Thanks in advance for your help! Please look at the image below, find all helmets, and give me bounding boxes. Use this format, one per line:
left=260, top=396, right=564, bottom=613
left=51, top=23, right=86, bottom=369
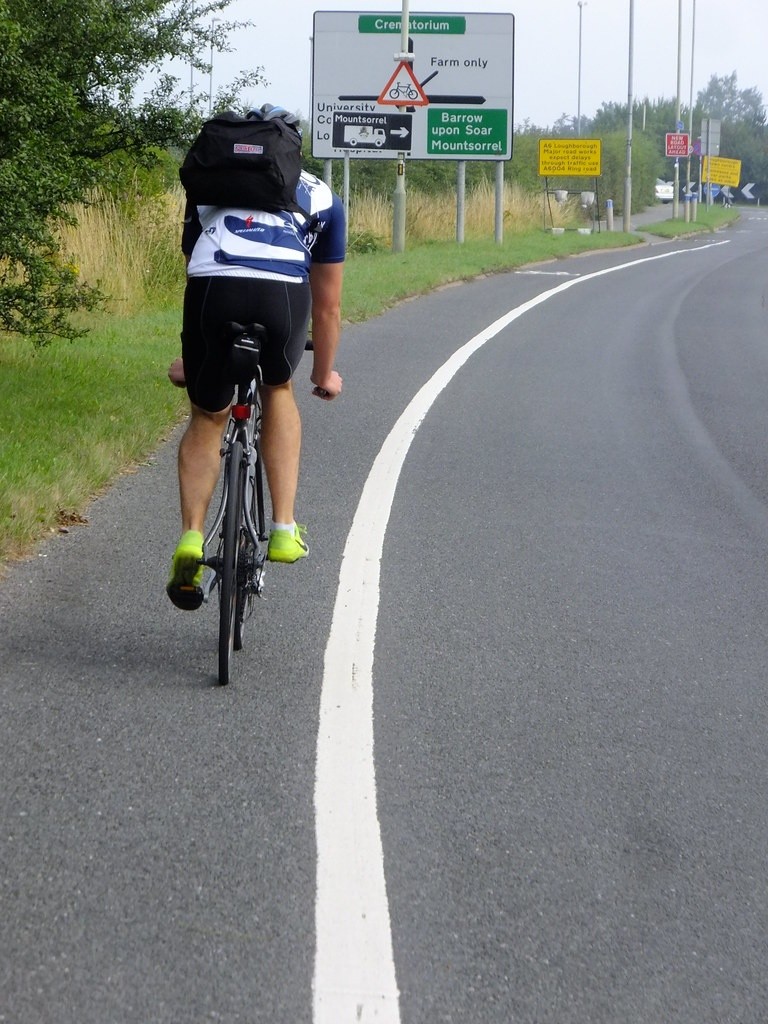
left=246, top=104, right=301, bottom=134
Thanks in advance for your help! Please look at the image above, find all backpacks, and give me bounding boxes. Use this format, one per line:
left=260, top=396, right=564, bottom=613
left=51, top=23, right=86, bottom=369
left=178, top=111, right=322, bottom=257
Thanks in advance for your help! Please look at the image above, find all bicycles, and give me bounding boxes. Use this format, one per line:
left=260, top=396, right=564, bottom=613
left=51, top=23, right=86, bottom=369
left=169, top=339, right=330, bottom=687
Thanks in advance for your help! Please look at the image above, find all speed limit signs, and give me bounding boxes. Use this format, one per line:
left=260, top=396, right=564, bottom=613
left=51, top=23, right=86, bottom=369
left=704, top=182, right=720, bottom=197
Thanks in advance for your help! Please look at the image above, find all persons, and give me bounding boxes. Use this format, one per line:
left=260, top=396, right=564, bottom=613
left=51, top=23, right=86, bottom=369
left=166, top=105, right=345, bottom=613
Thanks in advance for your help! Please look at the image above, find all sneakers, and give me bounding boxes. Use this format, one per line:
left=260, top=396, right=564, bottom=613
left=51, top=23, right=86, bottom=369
left=267, top=525, right=310, bottom=563
left=165, top=531, right=204, bottom=610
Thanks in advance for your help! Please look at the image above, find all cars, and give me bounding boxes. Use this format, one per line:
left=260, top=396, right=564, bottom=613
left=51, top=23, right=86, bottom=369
left=655, top=181, right=674, bottom=204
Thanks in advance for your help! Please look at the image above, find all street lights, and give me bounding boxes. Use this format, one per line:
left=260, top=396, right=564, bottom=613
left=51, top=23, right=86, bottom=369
left=578, top=1, right=587, bottom=138
